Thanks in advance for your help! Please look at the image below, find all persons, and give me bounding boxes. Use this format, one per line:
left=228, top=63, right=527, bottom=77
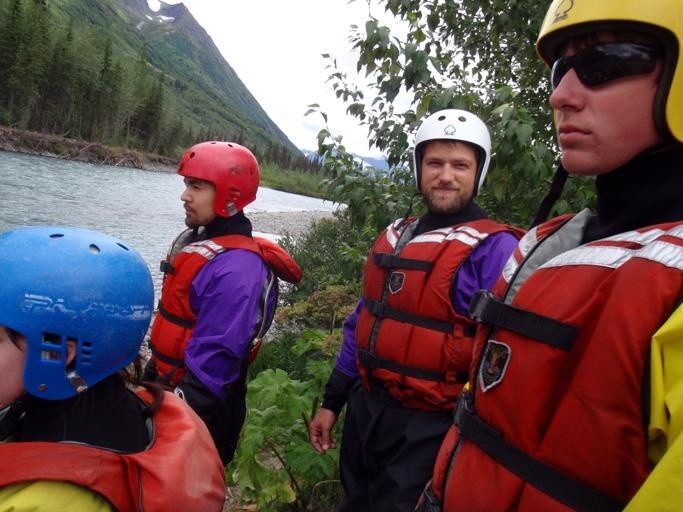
left=0, top=221, right=231, bottom=511
left=146, top=138, right=305, bottom=479
left=305, top=106, right=528, bottom=511
left=415, top=1, right=683, bottom=512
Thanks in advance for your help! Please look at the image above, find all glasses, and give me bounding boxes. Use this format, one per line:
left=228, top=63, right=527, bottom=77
left=544, top=39, right=665, bottom=90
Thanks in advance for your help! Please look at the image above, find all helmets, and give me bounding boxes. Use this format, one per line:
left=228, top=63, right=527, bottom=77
left=535, top=0, right=683, bottom=158
left=412, top=108, right=491, bottom=203
left=176, top=140, right=260, bottom=220
left=0, top=225, right=155, bottom=401
